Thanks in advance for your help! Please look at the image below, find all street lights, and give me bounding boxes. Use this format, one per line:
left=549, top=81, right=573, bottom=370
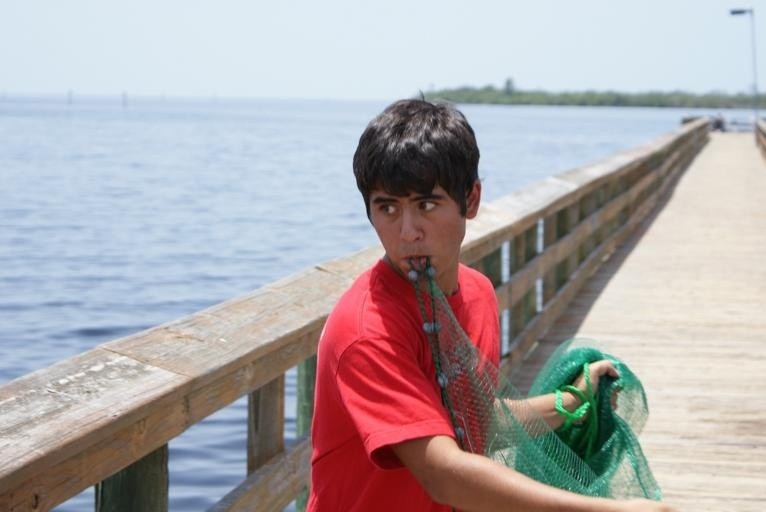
left=728, top=5, right=762, bottom=116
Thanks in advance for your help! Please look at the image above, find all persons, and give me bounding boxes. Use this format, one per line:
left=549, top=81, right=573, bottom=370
left=305, top=98, right=679, bottom=511
